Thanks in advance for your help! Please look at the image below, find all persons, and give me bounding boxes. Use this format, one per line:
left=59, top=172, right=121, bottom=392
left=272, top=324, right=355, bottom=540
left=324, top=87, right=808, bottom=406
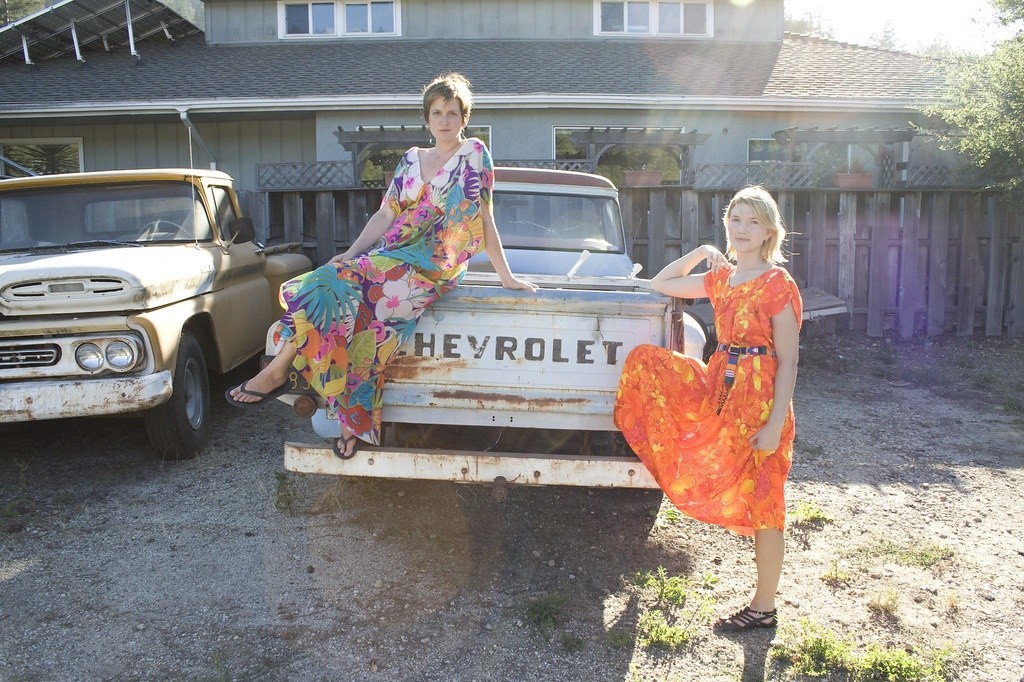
left=621, top=184, right=803, bottom=634
left=224, top=73, right=541, bottom=458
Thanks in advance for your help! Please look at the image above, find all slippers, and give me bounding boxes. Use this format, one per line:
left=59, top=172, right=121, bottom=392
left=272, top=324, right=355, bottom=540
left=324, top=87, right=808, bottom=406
left=333, top=433, right=357, bottom=459
left=224, top=373, right=292, bottom=409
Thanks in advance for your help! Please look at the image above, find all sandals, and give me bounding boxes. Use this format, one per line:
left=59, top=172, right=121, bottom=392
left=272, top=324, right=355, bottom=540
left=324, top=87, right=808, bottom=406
left=713, top=605, right=778, bottom=631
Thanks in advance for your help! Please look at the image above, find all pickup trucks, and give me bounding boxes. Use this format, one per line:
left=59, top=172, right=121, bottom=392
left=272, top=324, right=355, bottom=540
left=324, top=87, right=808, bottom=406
left=262, top=168, right=707, bottom=492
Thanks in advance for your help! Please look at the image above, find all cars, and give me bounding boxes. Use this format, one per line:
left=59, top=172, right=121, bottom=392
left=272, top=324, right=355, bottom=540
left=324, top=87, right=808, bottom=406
left=0, top=164, right=313, bottom=459
